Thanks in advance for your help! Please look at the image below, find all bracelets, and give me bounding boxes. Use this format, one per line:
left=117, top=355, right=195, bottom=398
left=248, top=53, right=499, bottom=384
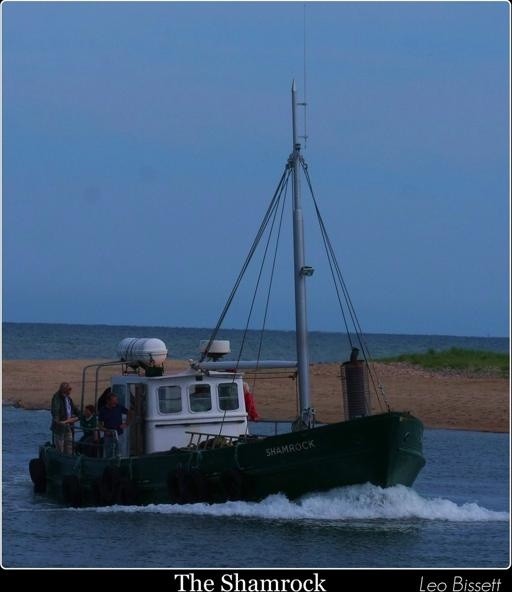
left=126, top=421, right=129, bottom=425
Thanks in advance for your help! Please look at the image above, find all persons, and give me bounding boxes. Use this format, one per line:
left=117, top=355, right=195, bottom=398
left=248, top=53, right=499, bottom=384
left=243, top=382, right=261, bottom=421
left=78, top=404, right=100, bottom=456
left=49, top=382, right=81, bottom=456
left=99, top=391, right=131, bottom=458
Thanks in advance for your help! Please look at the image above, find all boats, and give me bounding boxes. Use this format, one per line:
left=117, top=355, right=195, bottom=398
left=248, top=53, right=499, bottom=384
left=31, top=77, right=430, bottom=509
